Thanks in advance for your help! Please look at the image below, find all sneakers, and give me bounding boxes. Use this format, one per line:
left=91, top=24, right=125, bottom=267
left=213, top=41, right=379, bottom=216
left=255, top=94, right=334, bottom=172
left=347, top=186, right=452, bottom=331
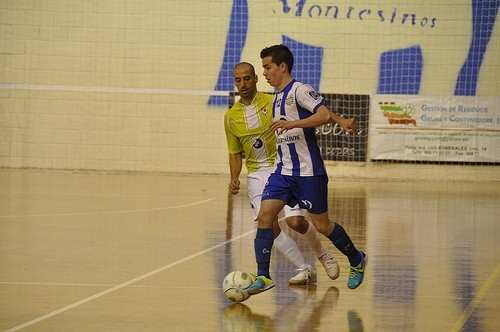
left=247, top=272, right=276, bottom=295
left=288, top=266, right=318, bottom=285
left=318, top=247, right=340, bottom=280
left=347, top=250, right=369, bottom=289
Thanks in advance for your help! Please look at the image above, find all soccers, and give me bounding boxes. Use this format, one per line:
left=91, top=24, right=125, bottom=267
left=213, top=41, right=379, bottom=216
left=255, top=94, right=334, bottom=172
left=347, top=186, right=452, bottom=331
left=223, top=303, right=251, bottom=330
left=222, top=270, right=253, bottom=303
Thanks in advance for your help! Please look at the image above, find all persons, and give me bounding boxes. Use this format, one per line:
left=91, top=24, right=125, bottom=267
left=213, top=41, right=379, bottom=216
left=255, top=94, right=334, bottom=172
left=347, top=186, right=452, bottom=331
left=224, top=62, right=357, bottom=284
left=248, top=44, right=368, bottom=295
